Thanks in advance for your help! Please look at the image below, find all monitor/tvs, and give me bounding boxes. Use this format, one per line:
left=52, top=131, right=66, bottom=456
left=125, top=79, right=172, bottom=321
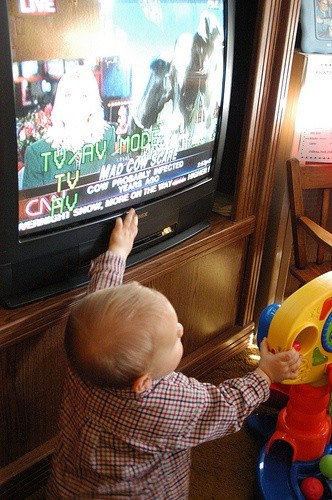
left=0, top=0, right=236, bottom=310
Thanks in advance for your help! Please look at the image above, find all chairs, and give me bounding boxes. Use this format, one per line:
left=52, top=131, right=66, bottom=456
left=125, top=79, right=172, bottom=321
left=286, top=157, right=332, bottom=285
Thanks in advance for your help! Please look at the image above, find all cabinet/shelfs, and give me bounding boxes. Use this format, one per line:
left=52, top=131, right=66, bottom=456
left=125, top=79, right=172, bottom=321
left=0, top=215, right=302, bottom=500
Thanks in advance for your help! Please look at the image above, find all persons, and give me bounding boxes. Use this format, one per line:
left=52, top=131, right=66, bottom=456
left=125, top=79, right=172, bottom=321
left=317, top=0, right=332, bottom=38
left=22, top=65, right=115, bottom=190
left=48, top=208, right=303, bottom=500
